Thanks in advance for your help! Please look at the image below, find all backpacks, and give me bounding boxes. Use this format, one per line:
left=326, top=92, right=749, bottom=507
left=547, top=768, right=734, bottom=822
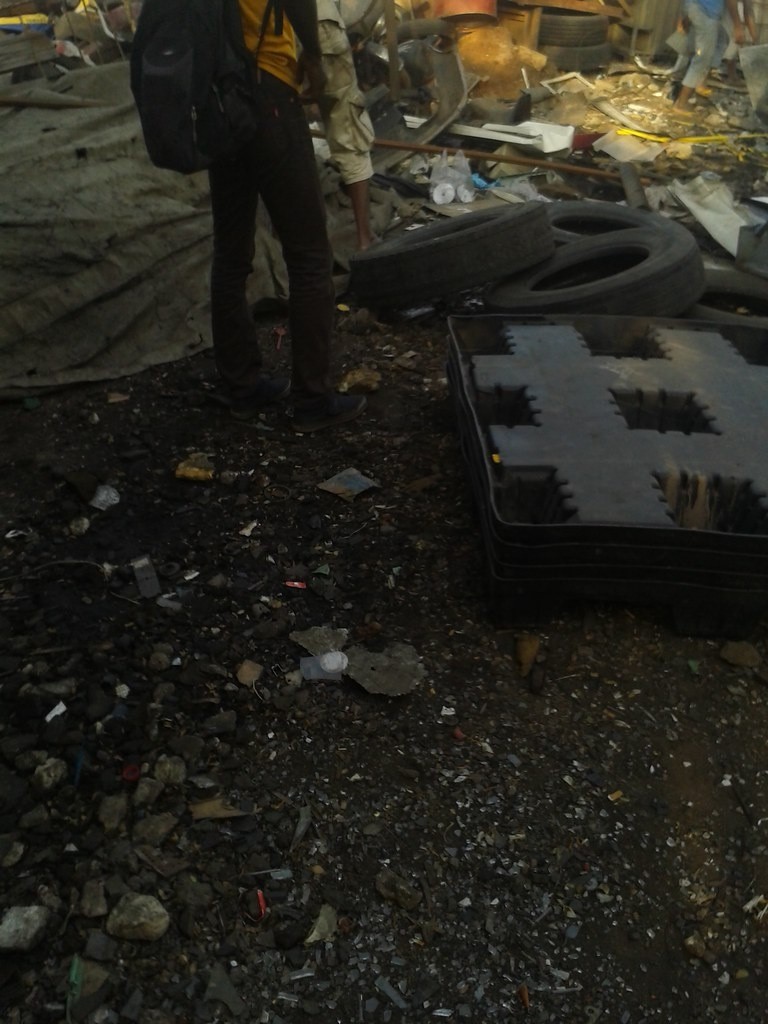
left=130, top=0, right=264, bottom=175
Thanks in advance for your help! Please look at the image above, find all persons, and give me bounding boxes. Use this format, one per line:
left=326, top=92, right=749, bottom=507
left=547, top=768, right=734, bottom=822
left=169, top=0, right=375, bottom=435
left=663, top=0, right=763, bottom=116
left=297, top=1, right=386, bottom=260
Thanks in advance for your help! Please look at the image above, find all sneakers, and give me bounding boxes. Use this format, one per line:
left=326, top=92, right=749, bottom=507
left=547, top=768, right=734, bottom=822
left=292, top=394, right=367, bottom=434
left=231, top=376, right=292, bottom=417
